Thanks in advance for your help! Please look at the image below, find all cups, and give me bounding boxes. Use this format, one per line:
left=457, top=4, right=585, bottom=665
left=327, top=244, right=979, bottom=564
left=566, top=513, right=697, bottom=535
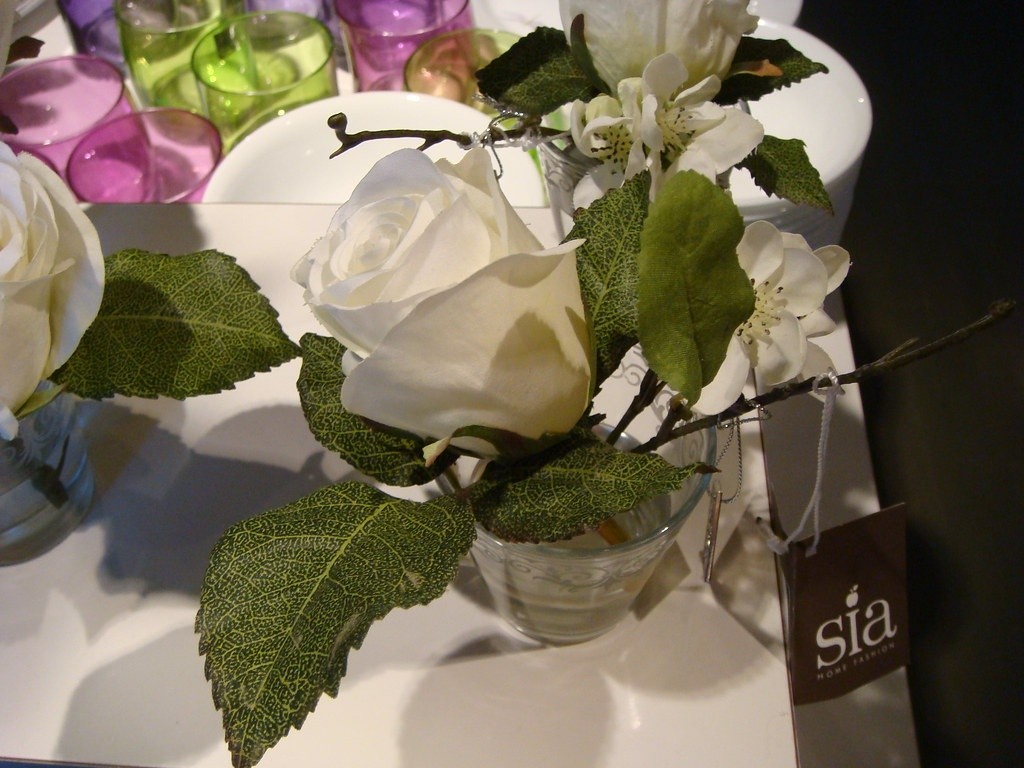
left=113, top=0, right=259, bottom=148
left=67, top=107, right=222, bottom=205
left=718, top=21, right=874, bottom=254
left=401, top=28, right=544, bottom=132
left=190, top=10, right=340, bottom=159
left=333, top=0, right=474, bottom=95
left=245, top=0, right=335, bottom=58
left=54, top=0, right=135, bottom=80
left=0, top=55, right=149, bottom=206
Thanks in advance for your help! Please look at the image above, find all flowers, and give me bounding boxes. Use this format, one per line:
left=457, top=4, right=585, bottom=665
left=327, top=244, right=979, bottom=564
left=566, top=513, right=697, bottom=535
left=0, top=137, right=305, bottom=509
left=188, top=3, right=1013, bottom=766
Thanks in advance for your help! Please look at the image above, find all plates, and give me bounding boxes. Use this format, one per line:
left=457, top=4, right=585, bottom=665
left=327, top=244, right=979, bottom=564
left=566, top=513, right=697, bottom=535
left=201, top=91, right=548, bottom=207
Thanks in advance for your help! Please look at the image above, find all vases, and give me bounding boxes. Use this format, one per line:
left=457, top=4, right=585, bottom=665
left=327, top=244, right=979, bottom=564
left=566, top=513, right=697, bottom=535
left=437, top=343, right=719, bottom=644
left=0, top=383, right=95, bottom=569
